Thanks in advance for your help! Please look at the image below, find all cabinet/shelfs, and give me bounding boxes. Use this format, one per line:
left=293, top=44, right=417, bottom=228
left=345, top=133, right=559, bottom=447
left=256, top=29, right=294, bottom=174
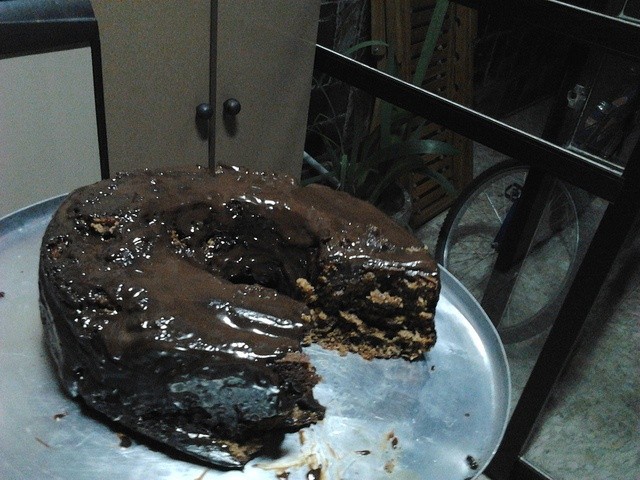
left=88, top=0, right=320, bottom=183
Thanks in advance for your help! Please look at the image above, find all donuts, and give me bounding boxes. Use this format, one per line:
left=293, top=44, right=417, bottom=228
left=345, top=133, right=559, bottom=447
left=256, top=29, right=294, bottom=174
left=43, top=166, right=441, bottom=469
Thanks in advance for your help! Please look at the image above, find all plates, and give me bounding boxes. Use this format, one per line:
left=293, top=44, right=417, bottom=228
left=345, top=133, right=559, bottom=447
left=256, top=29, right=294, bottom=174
left=0, top=191, right=512, bottom=479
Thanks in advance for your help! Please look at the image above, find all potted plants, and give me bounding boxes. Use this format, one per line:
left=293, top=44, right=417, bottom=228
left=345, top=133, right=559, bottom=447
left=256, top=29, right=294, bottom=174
left=307, top=0, right=464, bottom=237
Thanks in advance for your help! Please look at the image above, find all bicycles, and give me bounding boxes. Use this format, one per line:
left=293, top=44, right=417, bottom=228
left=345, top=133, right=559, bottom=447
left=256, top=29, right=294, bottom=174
left=435, top=78, right=628, bottom=344
